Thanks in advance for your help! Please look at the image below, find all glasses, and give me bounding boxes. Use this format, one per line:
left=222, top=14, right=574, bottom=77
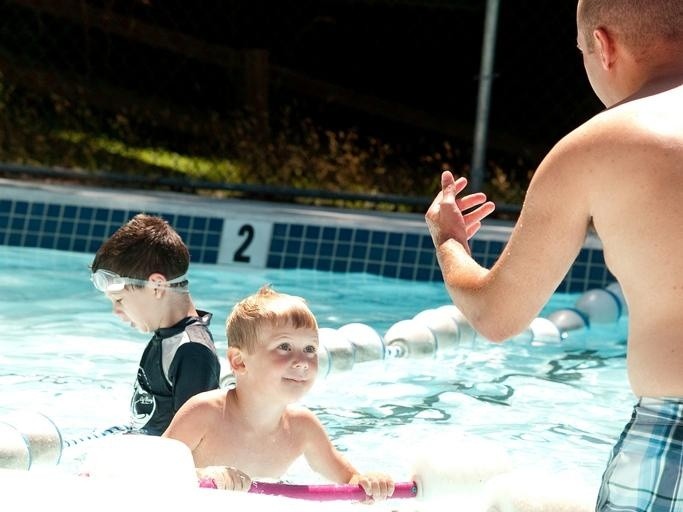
left=89, top=268, right=190, bottom=294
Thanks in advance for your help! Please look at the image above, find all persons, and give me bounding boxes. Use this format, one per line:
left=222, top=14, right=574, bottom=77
left=89, top=212, right=221, bottom=437
left=161, top=284, right=396, bottom=506
left=423, top=0, right=683, bottom=512
